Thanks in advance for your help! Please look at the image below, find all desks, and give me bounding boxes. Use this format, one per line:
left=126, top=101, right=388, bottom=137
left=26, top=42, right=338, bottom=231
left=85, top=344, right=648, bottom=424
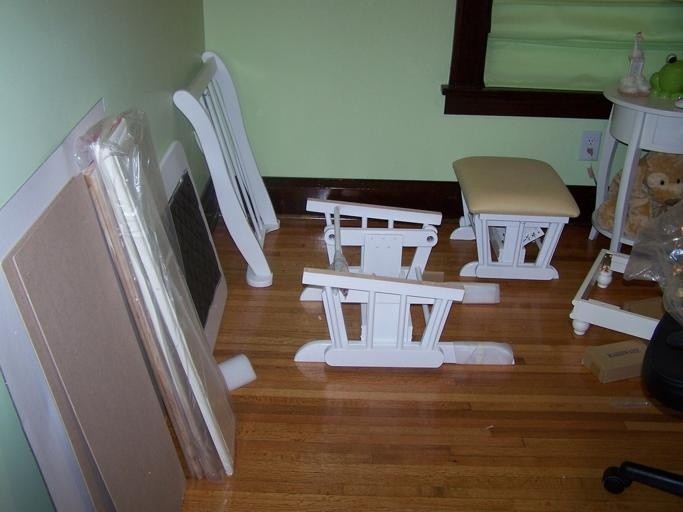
left=588, top=86, right=683, bottom=253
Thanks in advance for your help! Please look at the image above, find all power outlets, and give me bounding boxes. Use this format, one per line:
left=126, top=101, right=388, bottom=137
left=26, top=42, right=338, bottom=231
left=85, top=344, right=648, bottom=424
left=578, top=130, right=602, bottom=160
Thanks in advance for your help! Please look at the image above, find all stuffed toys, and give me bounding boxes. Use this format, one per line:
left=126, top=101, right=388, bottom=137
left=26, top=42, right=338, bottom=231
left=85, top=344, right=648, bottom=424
left=595, top=152, right=683, bottom=238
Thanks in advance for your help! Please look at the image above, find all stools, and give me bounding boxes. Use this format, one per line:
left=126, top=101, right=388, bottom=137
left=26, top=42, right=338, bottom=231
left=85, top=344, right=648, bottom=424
left=451, top=156, right=580, bottom=280
left=604, top=311, right=683, bottom=501
